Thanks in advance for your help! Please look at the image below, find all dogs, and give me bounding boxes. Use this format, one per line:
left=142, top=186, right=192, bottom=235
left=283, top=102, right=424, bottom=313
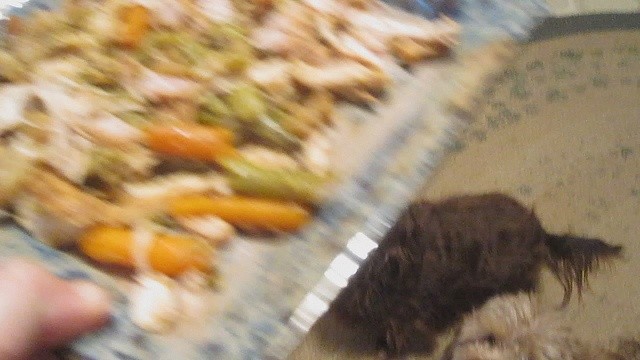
left=446, top=292, right=640, bottom=360
left=329, top=192, right=624, bottom=360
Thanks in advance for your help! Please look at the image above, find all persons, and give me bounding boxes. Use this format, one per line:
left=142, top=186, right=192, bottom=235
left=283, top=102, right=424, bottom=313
left=0, top=259, right=115, bottom=360
